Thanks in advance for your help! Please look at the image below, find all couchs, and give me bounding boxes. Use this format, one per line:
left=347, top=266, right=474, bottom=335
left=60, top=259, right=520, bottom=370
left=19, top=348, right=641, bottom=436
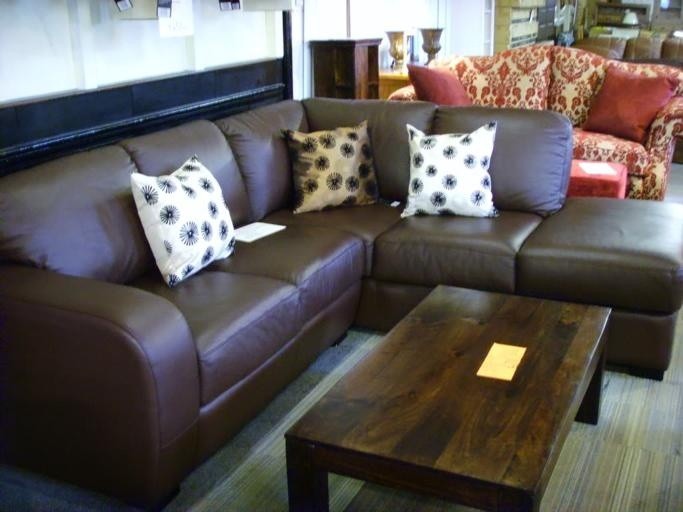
left=0, top=94, right=682, bottom=512
left=384, top=45, right=682, bottom=199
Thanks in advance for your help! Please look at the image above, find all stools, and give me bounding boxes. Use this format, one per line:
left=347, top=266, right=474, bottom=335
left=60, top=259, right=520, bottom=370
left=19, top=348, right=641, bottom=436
left=562, top=158, right=628, bottom=199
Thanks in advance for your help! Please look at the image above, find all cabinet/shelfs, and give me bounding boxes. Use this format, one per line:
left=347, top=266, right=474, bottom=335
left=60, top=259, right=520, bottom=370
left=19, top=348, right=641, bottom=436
left=306, top=36, right=384, bottom=102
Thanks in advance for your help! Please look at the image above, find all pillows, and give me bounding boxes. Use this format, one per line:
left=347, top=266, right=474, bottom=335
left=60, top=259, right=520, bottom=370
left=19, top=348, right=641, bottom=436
left=582, top=65, right=682, bottom=142
left=278, top=119, right=385, bottom=216
left=121, top=154, right=238, bottom=289
left=406, top=63, right=474, bottom=106
left=399, top=114, right=503, bottom=220
